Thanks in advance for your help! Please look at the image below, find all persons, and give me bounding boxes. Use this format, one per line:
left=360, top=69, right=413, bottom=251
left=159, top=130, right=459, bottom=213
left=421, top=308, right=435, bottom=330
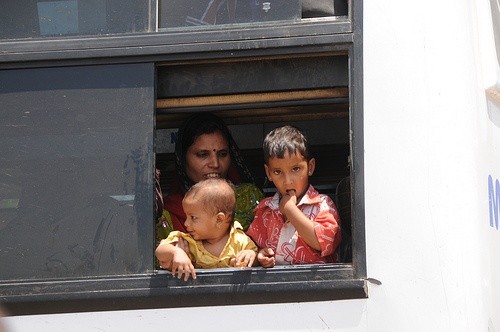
left=245, top=125, right=342, bottom=269
left=155, top=177, right=258, bottom=282
left=154, top=116, right=262, bottom=266
